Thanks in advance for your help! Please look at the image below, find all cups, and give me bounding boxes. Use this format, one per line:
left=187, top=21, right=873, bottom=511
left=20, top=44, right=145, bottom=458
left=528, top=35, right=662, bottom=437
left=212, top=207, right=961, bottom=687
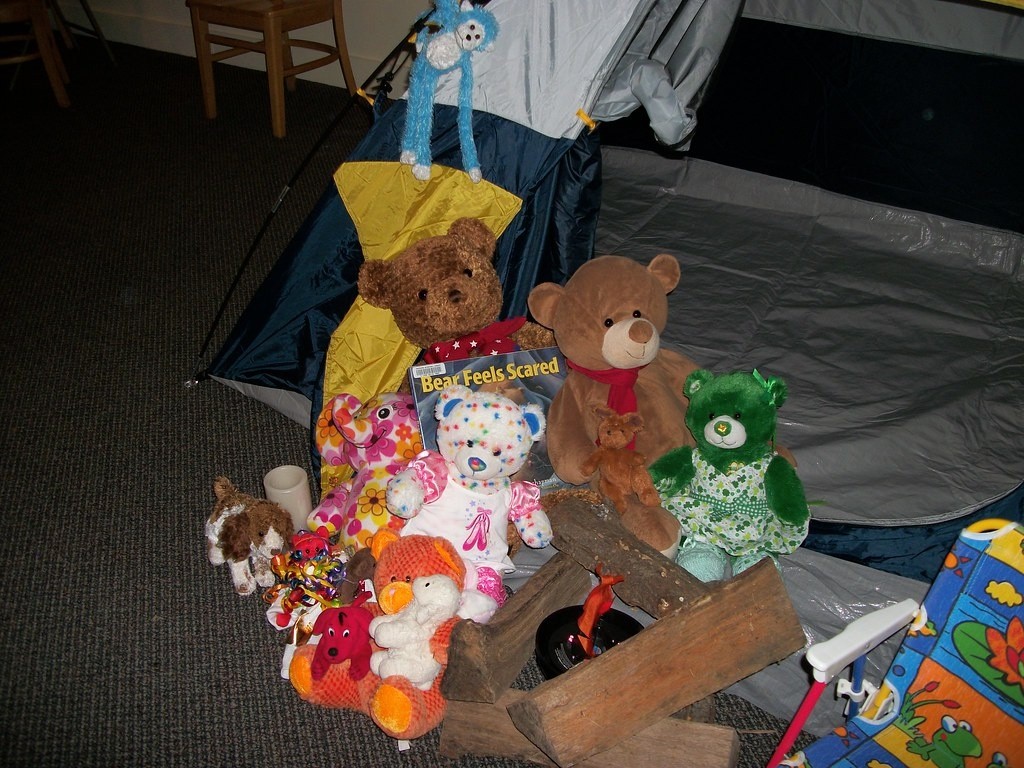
left=264, top=465, right=313, bottom=534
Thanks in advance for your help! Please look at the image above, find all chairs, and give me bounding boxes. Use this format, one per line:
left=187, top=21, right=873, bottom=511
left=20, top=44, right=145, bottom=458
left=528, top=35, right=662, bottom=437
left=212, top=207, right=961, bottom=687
left=764, top=516, right=1024, bottom=768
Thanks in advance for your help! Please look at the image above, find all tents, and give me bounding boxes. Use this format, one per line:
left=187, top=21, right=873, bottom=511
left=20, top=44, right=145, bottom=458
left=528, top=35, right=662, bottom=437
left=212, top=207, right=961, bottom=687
left=184, top=0, right=1024, bottom=737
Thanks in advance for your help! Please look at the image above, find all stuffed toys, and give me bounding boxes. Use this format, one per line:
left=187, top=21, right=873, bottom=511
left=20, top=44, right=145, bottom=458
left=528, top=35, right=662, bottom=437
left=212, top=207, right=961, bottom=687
left=399, top=0, right=499, bottom=183
left=386, top=385, right=553, bottom=625
left=528, top=254, right=795, bottom=551
left=645, top=369, right=811, bottom=583
left=357, top=217, right=558, bottom=395
left=580, top=403, right=661, bottom=516
left=204, top=476, right=294, bottom=596
left=262, top=394, right=466, bottom=740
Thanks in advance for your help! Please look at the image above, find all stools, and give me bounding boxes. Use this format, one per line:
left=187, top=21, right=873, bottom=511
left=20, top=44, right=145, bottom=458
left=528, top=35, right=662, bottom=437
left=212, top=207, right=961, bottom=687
left=0, top=0, right=118, bottom=108
left=186, top=0, right=357, bottom=138
